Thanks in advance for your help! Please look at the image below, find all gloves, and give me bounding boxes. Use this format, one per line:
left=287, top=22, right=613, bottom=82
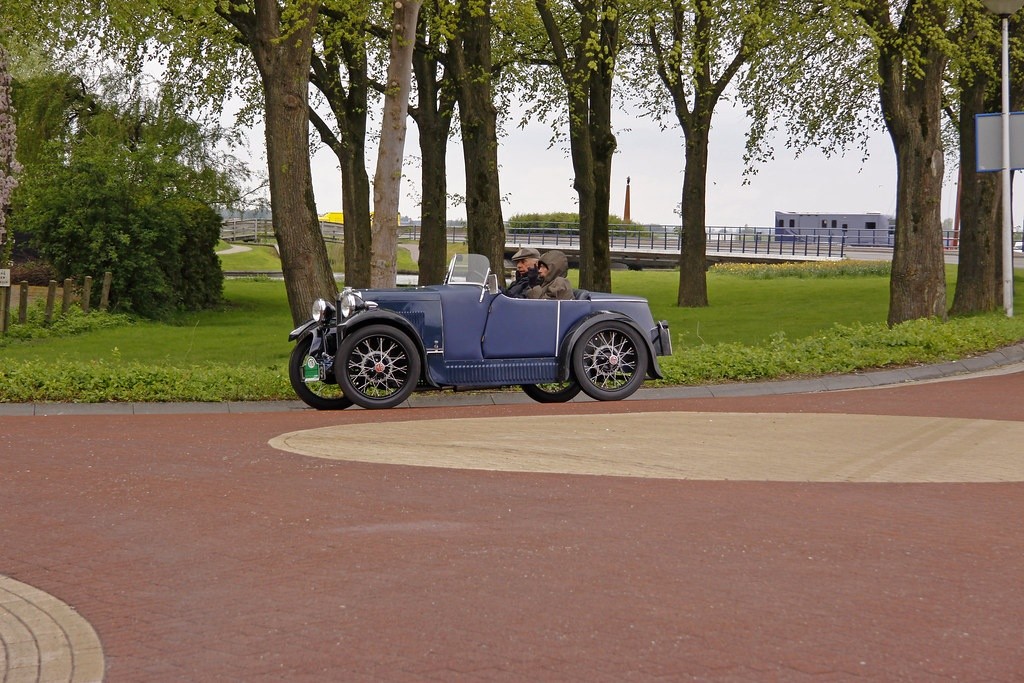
left=527, top=263, right=538, bottom=287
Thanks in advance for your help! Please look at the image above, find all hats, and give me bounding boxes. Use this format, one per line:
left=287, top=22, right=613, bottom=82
left=512, top=248, right=541, bottom=261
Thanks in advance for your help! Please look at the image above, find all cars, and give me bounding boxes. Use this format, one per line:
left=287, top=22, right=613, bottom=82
left=284, top=251, right=674, bottom=409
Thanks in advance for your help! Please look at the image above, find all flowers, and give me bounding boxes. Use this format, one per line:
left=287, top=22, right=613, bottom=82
left=0, top=42, right=24, bottom=270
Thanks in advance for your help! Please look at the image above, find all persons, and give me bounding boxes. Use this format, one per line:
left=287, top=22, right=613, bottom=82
left=488, top=248, right=540, bottom=298
left=521, top=250, right=575, bottom=300
